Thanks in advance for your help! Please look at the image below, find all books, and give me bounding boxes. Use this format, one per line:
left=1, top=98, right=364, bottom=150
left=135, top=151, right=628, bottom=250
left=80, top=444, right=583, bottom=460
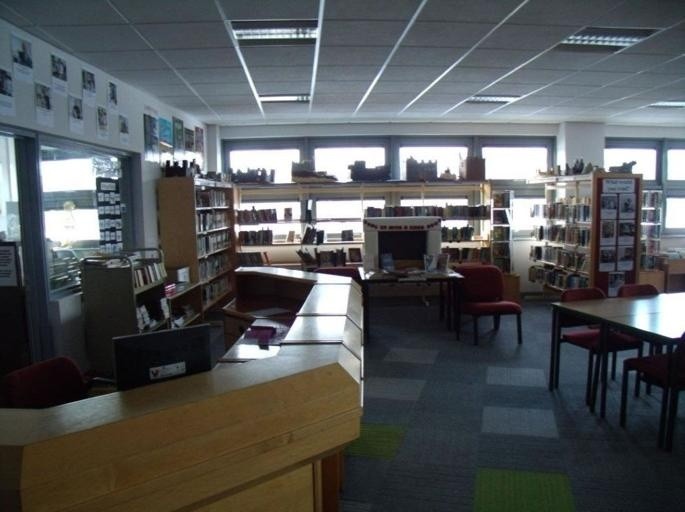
left=442, top=246, right=490, bottom=261
left=342, top=230, right=352, bottom=239
left=121, top=261, right=177, bottom=330
left=493, top=189, right=512, bottom=273
left=237, top=208, right=276, bottom=266
left=288, top=230, right=295, bottom=242
left=442, top=227, right=472, bottom=240
left=285, top=208, right=292, bottom=218
left=198, top=188, right=232, bottom=303
left=302, top=227, right=325, bottom=245
left=296, top=248, right=362, bottom=267
left=363, top=207, right=492, bottom=220
left=530, top=203, right=590, bottom=292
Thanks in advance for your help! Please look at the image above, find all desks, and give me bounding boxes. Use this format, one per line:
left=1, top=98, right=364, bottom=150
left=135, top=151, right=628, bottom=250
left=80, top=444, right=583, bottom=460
left=658, top=256, right=685, bottom=293
left=355, top=267, right=466, bottom=342
left=549, top=292, right=685, bottom=451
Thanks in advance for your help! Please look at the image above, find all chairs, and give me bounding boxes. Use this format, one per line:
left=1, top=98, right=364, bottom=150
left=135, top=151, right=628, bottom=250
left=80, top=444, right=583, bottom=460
left=619, top=331, right=685, bottom=454
left=610, top=283, right=674, bottom=396
left=453, top=264, right=523, bottom=345
left=315, top=267, right=370, bottom=341
left=0, top=355, right=117, bottom=409
left=554, top=286, right=643, bottom=406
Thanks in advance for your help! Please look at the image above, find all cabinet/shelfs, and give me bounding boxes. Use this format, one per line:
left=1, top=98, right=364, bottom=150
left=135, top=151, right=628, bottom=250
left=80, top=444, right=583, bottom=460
left=79, top=247, right=171, bottom=378
left=165, top=281, right=203, bottom=329
left=528, top=167, right=665, bottom=298
left=235, top=181, right=514, bottom=273
left=157, top=175, right=236, bottom=322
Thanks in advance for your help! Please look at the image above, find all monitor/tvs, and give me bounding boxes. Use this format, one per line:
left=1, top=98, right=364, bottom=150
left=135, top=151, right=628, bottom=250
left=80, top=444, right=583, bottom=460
left=111, top=318, right=227, bottom=392
